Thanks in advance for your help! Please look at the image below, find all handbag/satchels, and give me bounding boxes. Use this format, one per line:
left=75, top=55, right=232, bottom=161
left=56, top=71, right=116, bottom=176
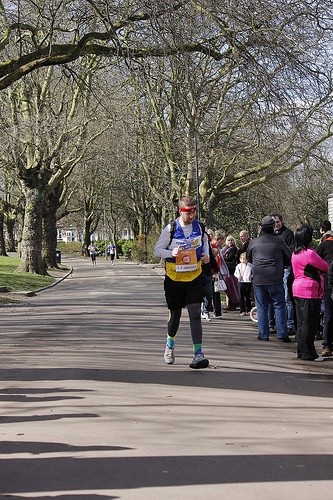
left=214, top=276, right=228, bottom=293
left=223, top=276, right=239, bottom=304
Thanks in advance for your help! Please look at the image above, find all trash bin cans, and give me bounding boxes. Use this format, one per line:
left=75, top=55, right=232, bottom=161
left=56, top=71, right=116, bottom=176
left=55, top=251, right=61, bottom=263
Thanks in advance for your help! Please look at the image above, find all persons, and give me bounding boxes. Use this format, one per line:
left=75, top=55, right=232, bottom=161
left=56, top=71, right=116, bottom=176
left=246, top=216, right=293, bottom=342
left=107, top=241, right=115, bottom=266
left=233, top=252, right=253, bottom=315
left=221, top=235, right=238, bottom=312
left=269, top=213, right=294, bottom=335
left=239, top=230, right=251, bottom=251
left=201, top=232, right=213, bottom=321
left=88, top=241, right=98, bottom=266
left=319, top=220, right=333, bottom=246
left=315, top=234, right=333, bottom=356
left=153, top=196, right=210, bottom=369
left=291, top=225, right=329, bottom=362
left=201, top=229, right=229, bottom=322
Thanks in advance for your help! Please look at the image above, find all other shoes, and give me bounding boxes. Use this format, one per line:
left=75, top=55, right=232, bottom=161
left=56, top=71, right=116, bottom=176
left=241, top=312, right=246, bottom=316
left=201, top=312, right=209, bottom=318
left=247, top=311, right=249, bottom=315
left=322, top=346, right=332, bottom=355
left=314, top=357, right=324, bottom=361
left=277, top=335, right=291, bottom=342
left=257, top=335, right=269, bottom=340
left=214, top=313, right=222, bottom=319
left=224, top=306, right=235, bottom=311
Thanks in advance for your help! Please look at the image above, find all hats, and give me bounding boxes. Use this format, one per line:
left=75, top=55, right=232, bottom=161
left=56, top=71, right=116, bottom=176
left=259, top=216, right=275, bottom=227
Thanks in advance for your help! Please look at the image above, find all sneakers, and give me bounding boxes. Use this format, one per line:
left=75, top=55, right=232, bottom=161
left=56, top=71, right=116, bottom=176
left=190, top=354, right=208, bottom=369
left=164, top=345, right=174, bottom=364
